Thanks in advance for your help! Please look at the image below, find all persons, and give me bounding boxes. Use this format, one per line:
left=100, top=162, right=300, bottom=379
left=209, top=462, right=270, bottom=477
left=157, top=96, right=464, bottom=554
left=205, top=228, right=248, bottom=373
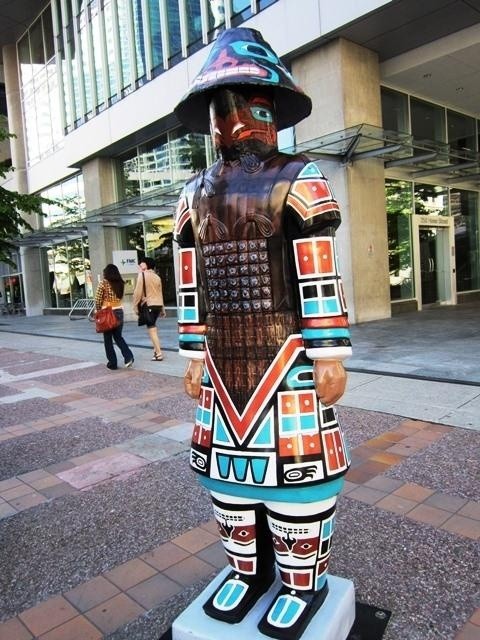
left=93, top=263, right=135, bottom=370
left=162, top=27, right=353, bottom=640
left=132, top=257, right=166, bottom=362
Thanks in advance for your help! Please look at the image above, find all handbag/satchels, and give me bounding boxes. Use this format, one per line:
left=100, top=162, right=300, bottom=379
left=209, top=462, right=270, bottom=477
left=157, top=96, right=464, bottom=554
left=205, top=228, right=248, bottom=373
left=138, top=300, right=151, bottom=326
left=93, top=307, right=119, bottom=333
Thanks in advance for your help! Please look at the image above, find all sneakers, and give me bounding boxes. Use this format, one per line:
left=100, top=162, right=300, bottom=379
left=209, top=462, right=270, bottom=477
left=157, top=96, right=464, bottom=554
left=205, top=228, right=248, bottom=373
left=125, top=359, right=134, bottom=368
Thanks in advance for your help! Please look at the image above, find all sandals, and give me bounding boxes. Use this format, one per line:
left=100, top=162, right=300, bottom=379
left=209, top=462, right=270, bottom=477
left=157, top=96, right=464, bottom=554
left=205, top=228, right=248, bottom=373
left=152, top=352, right=163, bottom=361
